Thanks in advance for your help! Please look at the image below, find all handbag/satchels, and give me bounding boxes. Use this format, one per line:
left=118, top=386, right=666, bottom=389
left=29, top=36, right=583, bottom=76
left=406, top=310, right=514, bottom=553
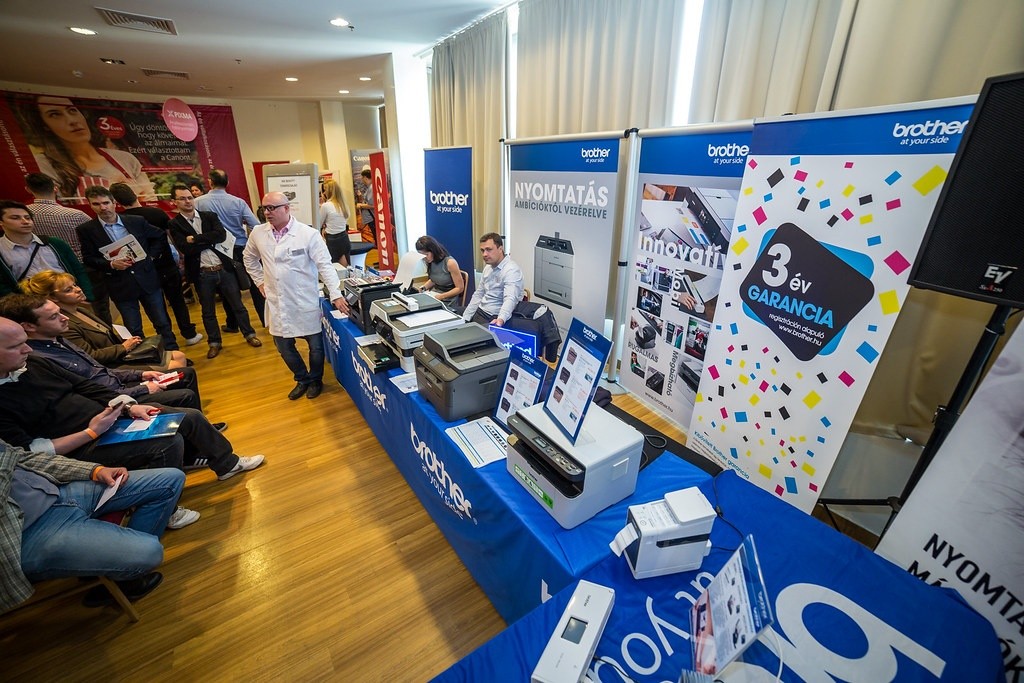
left=124, top=335, right=167, bottom=367
left=230, top=256, right=252, bottom=291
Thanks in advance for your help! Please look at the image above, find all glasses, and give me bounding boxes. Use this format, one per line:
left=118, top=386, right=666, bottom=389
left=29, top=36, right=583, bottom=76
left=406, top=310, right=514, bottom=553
left=175, top=196, right=194, bottom=201
left=262, top=204, right=288, bottom=212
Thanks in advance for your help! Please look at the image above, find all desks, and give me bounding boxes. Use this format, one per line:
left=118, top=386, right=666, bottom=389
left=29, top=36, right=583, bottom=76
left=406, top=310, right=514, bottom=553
left=321, top=293, right=724, bottom=581
left=427, top=470, right=1005, bottom=683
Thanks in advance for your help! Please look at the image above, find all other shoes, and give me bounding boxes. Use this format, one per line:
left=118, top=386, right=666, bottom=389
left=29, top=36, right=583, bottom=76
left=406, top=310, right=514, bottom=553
left=212, top=422, right=228, bottom=433
left=221, top=324, right=239, bottom=333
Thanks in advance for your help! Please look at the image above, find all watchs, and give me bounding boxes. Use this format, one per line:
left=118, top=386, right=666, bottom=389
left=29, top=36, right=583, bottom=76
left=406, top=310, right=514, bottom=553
left=192, top=236, right=195, bottom=243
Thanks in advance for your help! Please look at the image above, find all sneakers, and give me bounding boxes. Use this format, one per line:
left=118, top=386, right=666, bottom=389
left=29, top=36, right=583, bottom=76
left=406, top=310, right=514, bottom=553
left=184, top=457, right=210, bottom=468
left=219, top=454, right=265, bottom=480
left=168, top=508, right=200, bottom=528
left=185, top=333, right=204, bottom=345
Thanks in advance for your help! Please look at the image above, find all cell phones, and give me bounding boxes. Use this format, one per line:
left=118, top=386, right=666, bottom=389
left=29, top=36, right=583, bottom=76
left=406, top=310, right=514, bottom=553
left=112, top=401, right=123, bottom=410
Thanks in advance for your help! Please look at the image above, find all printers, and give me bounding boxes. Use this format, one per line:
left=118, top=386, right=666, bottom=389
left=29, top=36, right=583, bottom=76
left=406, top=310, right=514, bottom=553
left=675, top=361, right=704, bottom=405
left=553, top=346, right=577, bottom=403
left=533, top=232, right=574, bottom=310
left=342, top=280, right=512, bottom=422
left=639, top=184, right=737, bottom=271
left=501, top=368, right=518, bottom=412
left=635, top=325, right=656, bottom=349
left=506, top=399, right=645, bottom=529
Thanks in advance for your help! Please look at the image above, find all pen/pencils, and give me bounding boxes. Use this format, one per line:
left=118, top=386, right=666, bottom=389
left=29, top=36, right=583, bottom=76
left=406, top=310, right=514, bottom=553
left=159, top=372, right=184, bottom=384
left=132, top=409, right=161, bottom=419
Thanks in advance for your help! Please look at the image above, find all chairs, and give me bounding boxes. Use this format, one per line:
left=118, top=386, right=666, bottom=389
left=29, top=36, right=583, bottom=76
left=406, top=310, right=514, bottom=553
left=0, top=508, right=139, bottom=636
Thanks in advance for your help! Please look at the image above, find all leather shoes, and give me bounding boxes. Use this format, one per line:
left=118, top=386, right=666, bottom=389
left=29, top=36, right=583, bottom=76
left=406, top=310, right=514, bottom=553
left=205, top=344, right=222, bottom=359
left=84, top=572, right=164, bottom=607
left=288, top=381, right=308, bottom=399
left=245, top=335, right=261, bottom=347
left=309, top=381, right=325, bottom=398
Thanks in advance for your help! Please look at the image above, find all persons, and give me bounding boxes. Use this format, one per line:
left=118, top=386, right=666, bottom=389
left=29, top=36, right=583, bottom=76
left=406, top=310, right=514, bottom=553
left=416, top=235, right=464, bottom=309
left=0, top=201, right=94, bottom=305
left=0, top=270, right=265, bottom=606
left=22, top=172, right=114, bottom=335
left=641, top=290, right=657, bottom=310
left=631, top=352, right=641, bottom=371
left=74, top=181, right=203, bottom=366
left=674, top=292, right=697, bottom=309
left=243, top=192, right=350, bottom=400
left=21, top=93, right=160, bottom=201
left=461, top=232, right=524, bottom=330
left=356, top=169, right=376, bottom=249
left=317, top=181, right=351, bottom=269
left=166, top=168, right=261, bottom=359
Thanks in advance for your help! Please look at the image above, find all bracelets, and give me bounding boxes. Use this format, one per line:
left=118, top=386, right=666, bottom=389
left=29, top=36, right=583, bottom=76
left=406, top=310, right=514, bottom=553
left=122, top=402, right=135, bottom=417
left=93, top=465, right=106, bottom=481
left=83, top=428, right=98, bottom=439
left=420, top=286, right=426, bottom=291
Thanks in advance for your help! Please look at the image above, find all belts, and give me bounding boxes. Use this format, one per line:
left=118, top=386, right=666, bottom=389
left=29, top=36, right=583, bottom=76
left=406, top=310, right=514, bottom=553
left=199, top=262, right=224, bottom=272
